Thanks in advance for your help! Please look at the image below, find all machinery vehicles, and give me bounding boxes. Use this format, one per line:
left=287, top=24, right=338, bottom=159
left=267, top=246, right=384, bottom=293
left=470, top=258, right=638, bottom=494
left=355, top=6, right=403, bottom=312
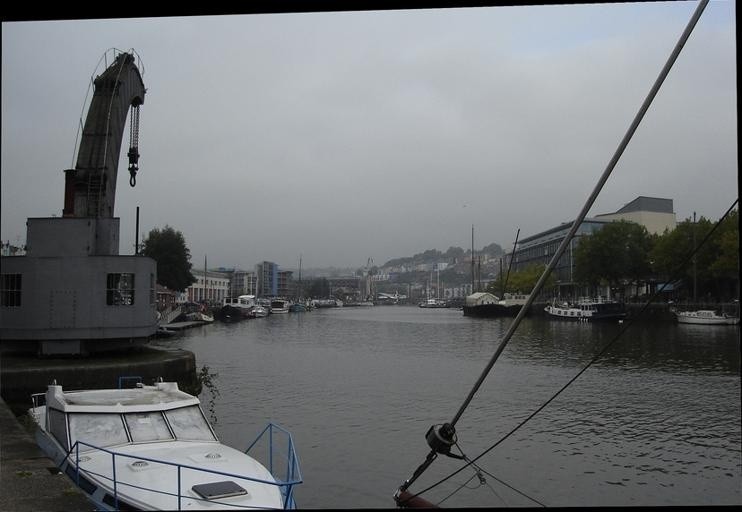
left=0, top=47, right=159, bottom=358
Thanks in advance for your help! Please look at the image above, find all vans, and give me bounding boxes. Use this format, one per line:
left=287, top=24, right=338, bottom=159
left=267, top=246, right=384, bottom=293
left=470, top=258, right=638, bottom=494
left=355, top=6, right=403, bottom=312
left=223, top=296, right=254, bottom=307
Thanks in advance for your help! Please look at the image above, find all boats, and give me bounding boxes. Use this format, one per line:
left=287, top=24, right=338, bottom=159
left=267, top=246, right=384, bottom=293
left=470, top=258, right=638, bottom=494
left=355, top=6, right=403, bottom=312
left=460, top=291, right=741, bottom=326
left=251, top=300, right=303, bottom=317
left=27, top=375, right=304, bottom=512
left=418, top=298, right=449, bottom=308
